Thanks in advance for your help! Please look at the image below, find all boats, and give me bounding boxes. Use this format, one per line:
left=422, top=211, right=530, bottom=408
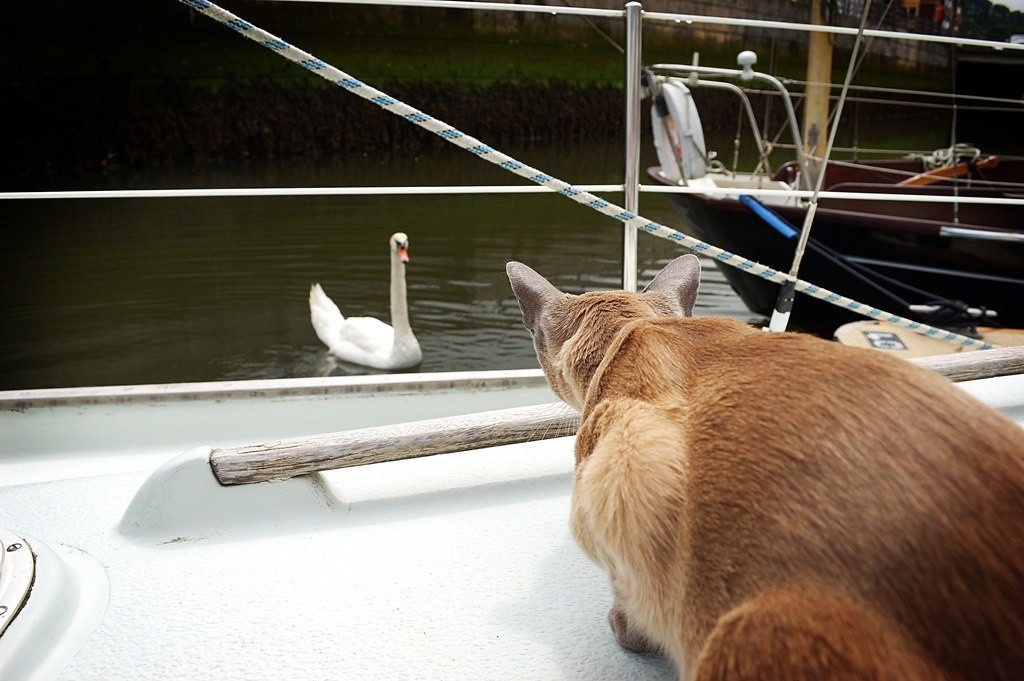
left=646, top=48, right=1023, bottom=340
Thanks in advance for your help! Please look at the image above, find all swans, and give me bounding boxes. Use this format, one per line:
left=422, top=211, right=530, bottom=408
left=308, top=232, right=424, bottom=369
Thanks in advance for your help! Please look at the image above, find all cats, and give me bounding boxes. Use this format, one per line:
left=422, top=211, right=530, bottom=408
left=506, top=255, right=1024, bottom=681
